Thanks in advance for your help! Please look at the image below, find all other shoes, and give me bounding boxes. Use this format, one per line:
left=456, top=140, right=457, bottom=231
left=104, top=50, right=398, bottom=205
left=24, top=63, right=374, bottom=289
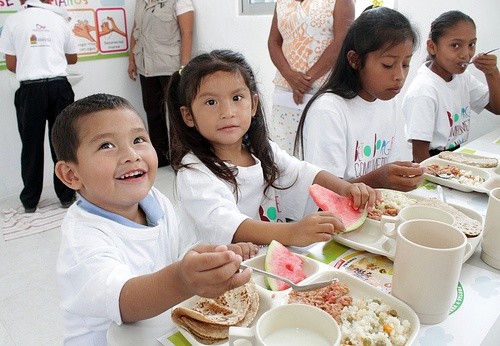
left=25, top=207, right=36, bottom=213
left=61, top=199, right=77, bottom=209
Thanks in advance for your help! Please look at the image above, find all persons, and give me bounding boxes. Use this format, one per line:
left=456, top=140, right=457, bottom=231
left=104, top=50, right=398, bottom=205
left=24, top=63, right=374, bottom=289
left=0, top=0, right=80, bottom=213
left=128, top=0, right=196, bottom=168
left=52, top=94, right=260, bottom=346
left=404, top=11, right=500, bottom=164
left=268, top=0, right=355, bottom=160
left=165, top=47, right=381, bottom=254
left=294, top=0, right=426, bottom=193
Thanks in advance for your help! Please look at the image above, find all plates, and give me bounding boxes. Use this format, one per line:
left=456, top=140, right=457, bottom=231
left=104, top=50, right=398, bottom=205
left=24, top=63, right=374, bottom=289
left=171, top=252, right=420, bottom=346
left=416, top=151, right=500, bottom=195
left=331, top=188, right=485, bottom=264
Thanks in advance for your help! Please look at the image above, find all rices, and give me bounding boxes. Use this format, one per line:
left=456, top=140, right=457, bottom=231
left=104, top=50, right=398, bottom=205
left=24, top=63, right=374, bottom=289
left=338, top=299, right=411, bottom=346
left=376, top=188, right=411, bottom=211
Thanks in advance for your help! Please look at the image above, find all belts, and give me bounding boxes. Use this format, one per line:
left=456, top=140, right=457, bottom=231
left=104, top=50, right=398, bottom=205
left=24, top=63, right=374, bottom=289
left=22, top=77, right=66, bottom=85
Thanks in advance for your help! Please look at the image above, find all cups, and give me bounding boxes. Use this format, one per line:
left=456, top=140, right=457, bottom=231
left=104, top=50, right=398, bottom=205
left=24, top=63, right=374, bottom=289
left=480, top=187, right=500, bottom=270
left=381, top=205, right=456, bottom=255
left=228, top=303, right=342, bottom=346
left=390, top=219, right=465, bottom=325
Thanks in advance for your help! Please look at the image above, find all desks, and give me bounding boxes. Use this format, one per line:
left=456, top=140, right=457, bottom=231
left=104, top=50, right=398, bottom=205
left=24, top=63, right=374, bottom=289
left=105, top=127, right=500, bottom=345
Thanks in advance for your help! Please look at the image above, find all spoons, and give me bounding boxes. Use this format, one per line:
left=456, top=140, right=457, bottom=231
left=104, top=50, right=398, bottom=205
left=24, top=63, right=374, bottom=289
left=458, top=48, right=500, bottom=67
left=238, top=264, right=337, bottom=292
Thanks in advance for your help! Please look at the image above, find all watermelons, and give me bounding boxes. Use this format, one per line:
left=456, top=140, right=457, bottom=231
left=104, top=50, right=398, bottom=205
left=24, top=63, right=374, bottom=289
left=264, top=240, right=307, bottom=291
left=308, top=183, right=369, bottom=232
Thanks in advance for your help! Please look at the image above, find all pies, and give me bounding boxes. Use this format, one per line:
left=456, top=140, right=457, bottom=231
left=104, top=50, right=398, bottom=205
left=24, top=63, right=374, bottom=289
left=171, top=276, right=259, bottom=342
left=418, top=199, right=482, bottom=237
left=439, top=151, right=498, bottom=167
